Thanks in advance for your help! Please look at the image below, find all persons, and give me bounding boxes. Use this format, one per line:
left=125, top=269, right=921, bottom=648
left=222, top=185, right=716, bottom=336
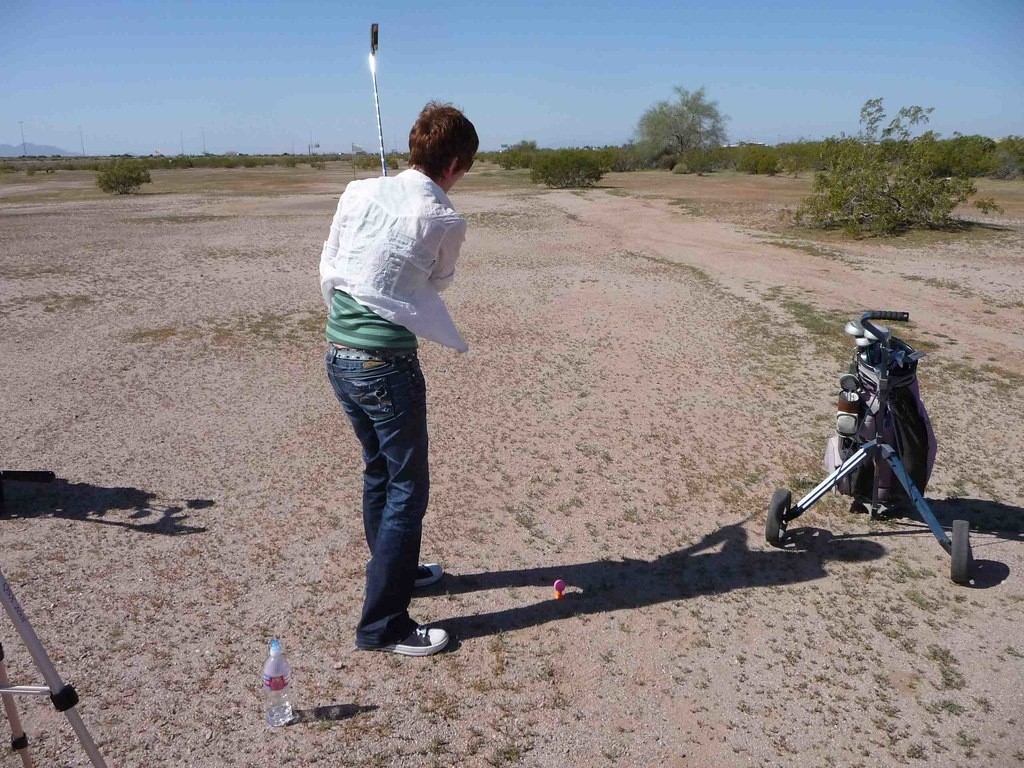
left=320, top=102, right=479, bottom=656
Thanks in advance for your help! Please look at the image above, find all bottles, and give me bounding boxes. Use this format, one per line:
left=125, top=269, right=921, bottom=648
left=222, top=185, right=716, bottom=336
left=262, top=640, right=293, bottom=727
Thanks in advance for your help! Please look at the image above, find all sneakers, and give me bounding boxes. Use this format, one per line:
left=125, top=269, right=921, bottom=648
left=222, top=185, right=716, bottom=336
left=373, top=624, right=449, bottom=657
left=414, top=564, right=443, bottom=586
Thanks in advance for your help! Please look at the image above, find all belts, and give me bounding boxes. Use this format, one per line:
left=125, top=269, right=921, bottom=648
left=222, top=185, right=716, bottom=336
left=327, top=342, right=411, bottom=361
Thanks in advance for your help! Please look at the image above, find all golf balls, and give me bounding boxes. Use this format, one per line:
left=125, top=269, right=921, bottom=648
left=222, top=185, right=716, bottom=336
left=554, top=579, right=566, bottom=591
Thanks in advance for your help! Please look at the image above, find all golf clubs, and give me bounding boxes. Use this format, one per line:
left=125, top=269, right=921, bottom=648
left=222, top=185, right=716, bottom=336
left=370, top=22, right=388, bottom=177
left=837, top=319, right=906, bottom=403
